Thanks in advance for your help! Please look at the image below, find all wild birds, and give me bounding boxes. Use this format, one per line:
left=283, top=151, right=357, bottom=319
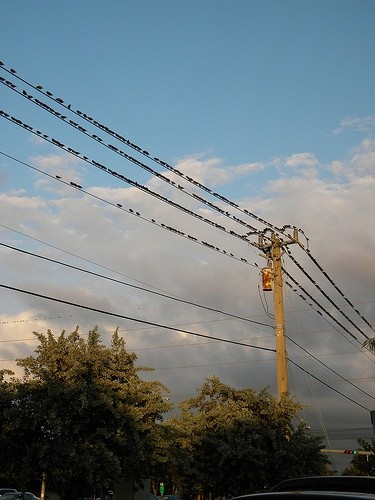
left=0, top=60, right=375, bottom=343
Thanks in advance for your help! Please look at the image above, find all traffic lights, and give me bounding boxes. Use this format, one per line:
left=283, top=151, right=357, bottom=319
left=344, top=450, right=358, bottom=455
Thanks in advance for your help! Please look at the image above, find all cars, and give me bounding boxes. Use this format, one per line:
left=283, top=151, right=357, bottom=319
left=0, top=488, right=18, bottom=498
left=0, top=492, right=41, bottom=500
